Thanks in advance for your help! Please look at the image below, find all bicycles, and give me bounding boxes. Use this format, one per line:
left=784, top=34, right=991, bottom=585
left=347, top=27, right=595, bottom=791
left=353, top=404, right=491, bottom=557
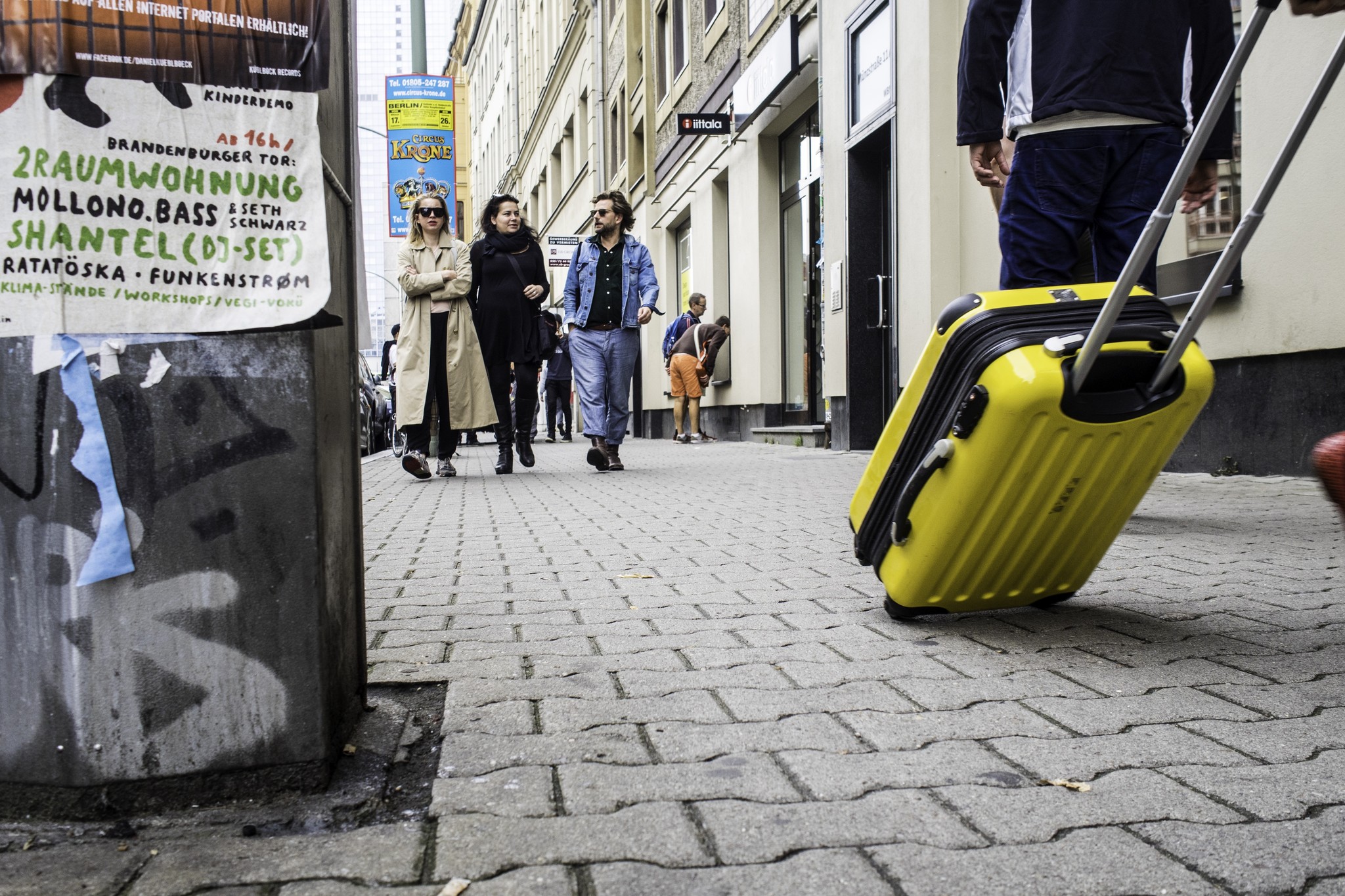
left=391, top=365, right=410, bottom=458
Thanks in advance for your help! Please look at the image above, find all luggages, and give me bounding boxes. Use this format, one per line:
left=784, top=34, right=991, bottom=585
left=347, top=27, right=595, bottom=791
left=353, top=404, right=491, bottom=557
left=848, top=0, right=1345, bottom=621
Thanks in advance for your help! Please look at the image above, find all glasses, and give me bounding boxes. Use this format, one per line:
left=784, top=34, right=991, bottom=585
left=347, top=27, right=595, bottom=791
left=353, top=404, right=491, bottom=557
left=696, top=303, right=706, bottom=308
left=590, top=209, right=619, bottom=217
left=492, top=194, right=514, bottom=204
left=417, top=207, right=444, bottom=217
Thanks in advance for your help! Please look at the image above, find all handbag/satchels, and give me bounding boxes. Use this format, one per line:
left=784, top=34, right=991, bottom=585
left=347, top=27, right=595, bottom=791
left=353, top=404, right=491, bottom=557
left=575, top=242, right=582, bottom=313
left=696, top=350, right=711, bottom=387
left=539, top=310, right=558, bottom=360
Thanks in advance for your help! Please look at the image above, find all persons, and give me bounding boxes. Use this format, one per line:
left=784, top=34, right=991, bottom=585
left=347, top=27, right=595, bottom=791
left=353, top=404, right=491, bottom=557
left=382, top=324, right=403, bottom=449
left=956, top=0, right=1236, bottom=295
left=466, top=194, right=550, bottom=475
left=662, top=292, right=730, bottom=443
left=562, top=190, right=666, bottom=472
left=396, top=192, right=499, bottom=479
left=455, top=314, right=578, bottom=448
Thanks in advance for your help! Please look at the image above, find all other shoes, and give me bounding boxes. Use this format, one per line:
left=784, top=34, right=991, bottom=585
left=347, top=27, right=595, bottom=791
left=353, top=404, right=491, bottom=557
left=1311, top=431, right=1345, bottom=512
left=698, top=428, right=718, bottom=442
left=456, top=424, right=572, bottom=447
left=673, top=429, right=678, bottom=443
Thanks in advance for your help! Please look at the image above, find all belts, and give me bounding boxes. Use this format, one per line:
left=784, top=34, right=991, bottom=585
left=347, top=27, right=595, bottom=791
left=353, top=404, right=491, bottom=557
left=588, top=323, right=621, bottom=330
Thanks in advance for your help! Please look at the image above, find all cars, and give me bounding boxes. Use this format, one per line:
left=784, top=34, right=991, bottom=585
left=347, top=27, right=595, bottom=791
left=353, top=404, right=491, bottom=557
left=357, top=351, right=393, bottom=456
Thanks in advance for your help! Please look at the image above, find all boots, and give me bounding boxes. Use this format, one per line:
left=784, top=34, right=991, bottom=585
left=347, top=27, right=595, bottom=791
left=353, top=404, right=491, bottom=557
left=605, top=443, right=624, bottom=470
left=492, top=404, right=513, bottom=474
left=587, top=437, right=610, bottom=471
left=515, top=394, right=536, bottom=467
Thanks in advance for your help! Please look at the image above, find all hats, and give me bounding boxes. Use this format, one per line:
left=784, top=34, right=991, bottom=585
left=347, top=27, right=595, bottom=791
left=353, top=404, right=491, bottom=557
left=553, top=313, right=562, bottom=326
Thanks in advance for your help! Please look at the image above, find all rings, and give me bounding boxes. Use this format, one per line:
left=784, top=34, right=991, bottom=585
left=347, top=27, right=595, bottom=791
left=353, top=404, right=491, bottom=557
left=534, top=291, right=536, bottom=294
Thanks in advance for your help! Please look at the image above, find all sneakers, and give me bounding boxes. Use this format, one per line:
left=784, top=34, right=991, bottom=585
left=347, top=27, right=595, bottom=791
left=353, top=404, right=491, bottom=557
left=676, top=434, right=692, bottom=444
left=402, top=449, right=432, bottom=479
left=691, top=433, right=713, bottom=443
left=436, top=457, right=457, bottom=477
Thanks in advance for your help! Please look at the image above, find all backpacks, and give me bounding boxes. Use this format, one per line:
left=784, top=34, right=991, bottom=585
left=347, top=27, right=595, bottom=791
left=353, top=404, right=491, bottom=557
left=662, top=313, right=695, bottom=359
